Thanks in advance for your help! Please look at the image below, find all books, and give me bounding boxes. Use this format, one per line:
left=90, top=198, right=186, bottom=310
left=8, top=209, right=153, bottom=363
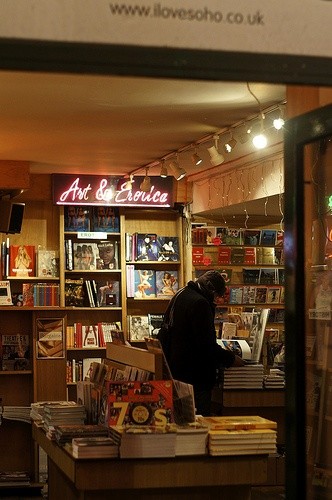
left=0, top=205, right=284, bottom=481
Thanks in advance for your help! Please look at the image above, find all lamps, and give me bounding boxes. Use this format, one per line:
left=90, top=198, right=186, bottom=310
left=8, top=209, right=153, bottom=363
left=139, top=166, right=152, bottom=192
left=191, top=142, right=203, bottom=166
left=122, top=172, right=134, bottom=190
left=168, top=152, right=186, bottom=181
left=159, top=158, right=168, bottom=178
left=207, top=135, right=225, bottom=166
left=224, top=104, right=286, bottom=153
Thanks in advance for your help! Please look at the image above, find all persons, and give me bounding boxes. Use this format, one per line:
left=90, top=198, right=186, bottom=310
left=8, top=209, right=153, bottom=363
left=66, top=206, right=75, bottom=228
left=96, top=242, right=117, bottom=270
left=158, top=270, right=247, bottom=417
left=107, top=208, right=116, bottom=229
left=13, top=246, right=32, bottom=277
left=96, top=206, right=106, bottom=229
left=75, top=204, right=85, bottom=227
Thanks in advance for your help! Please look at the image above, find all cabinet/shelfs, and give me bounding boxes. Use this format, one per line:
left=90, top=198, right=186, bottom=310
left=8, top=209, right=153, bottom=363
left=0, top=205, right=286, bottom=487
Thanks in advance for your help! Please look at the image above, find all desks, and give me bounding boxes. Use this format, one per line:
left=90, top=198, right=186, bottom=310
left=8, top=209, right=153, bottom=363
left=31, top=420, right=267, bottom=500
left=211, top=387, right=286, bottom=486
